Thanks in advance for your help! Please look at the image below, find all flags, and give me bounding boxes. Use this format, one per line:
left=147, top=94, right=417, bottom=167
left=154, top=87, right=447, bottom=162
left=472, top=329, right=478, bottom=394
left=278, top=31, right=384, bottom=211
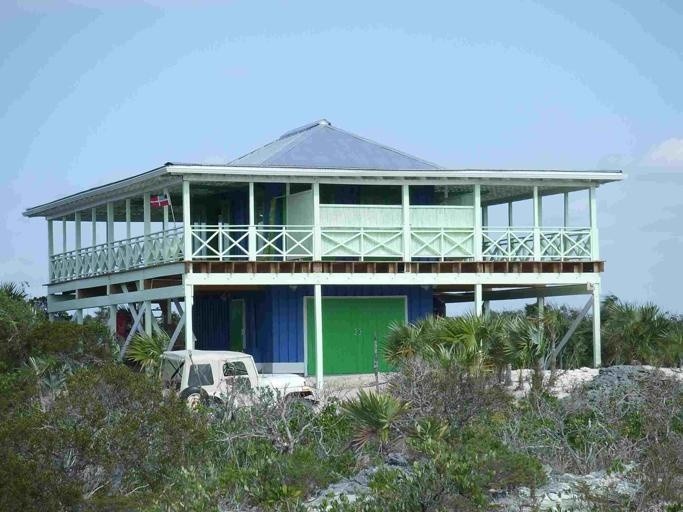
left=150, top=193, right=170, bottom=208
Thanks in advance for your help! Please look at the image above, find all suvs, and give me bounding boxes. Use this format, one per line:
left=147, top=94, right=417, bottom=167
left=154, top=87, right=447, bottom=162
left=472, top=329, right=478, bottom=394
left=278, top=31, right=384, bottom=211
left=157, top=348, right=320, bottom=428
